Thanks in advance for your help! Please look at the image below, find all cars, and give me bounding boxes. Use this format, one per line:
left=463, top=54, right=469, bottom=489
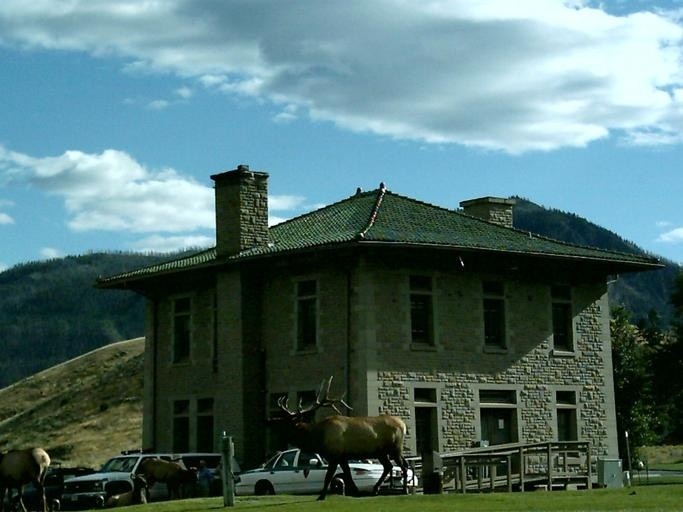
left=4, top=466, right=101, bottom=512
left=233, top=446, right=420, bottom=495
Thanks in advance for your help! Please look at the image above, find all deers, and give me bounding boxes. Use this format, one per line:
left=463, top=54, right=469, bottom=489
left=0, top=448, right=51, bottom=512
left=104, top=473, right=147, bottom=508
left=140, top=458, right=199, bottom=500
left=265, top=376, right=409, bottom=500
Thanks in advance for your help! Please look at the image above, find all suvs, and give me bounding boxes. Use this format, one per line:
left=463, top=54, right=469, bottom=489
left=61, top=449, right=242, bottom=509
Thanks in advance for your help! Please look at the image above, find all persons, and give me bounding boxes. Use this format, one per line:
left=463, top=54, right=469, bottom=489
left=216, top=458, right=222, bottom=497
left=170, top=453, right=194, bottom=497
left=196, top=460, right=215, bottom=497
